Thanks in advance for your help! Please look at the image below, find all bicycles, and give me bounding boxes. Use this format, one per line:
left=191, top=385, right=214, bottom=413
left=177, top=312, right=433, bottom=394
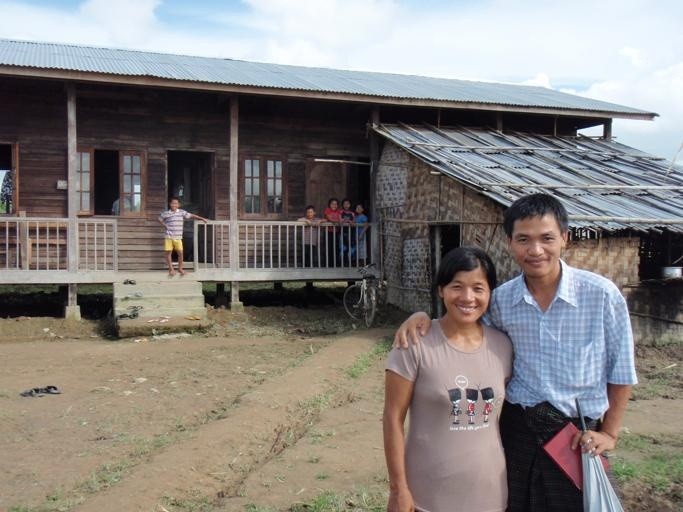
left=343, top=263, right=376, bottom=327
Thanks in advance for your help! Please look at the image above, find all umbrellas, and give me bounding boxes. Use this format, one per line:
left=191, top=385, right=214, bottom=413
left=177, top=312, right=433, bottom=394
left=575, top=397, right=625, bottom=511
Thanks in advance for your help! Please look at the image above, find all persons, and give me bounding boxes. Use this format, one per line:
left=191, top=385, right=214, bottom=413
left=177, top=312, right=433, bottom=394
left=321, top=197, right=341, bottom=267
left=349, top=203, right=369, bottom=268
left=155, top=196, right=208, bottom=277
left=380, top=246, right=517, bottom=512
left=390, top=192, right=641, bottom=511
left=295, top=205, right=328, bottom=267
left=110, top=194, right=132, bottom=216
left=336, top=198, right=356, bottom=267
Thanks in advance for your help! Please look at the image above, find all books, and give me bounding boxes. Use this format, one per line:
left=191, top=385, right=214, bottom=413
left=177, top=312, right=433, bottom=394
left=541, top=421, right=608, bottom=491
